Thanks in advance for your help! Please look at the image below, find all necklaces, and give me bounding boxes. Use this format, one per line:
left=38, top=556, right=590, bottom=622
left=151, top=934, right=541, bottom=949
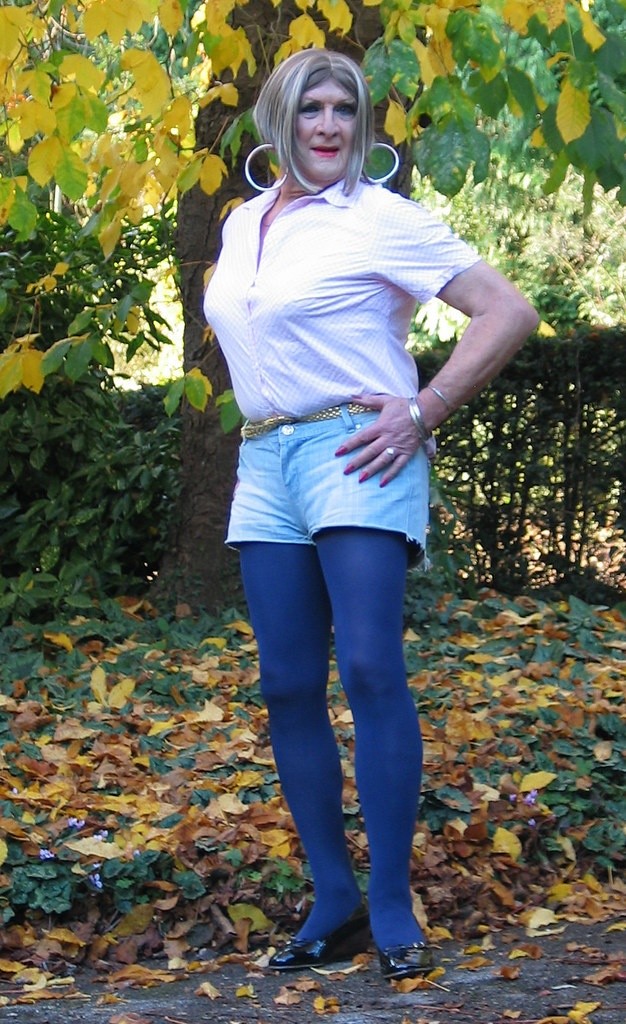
left=261, top=218, right=271, bottom=227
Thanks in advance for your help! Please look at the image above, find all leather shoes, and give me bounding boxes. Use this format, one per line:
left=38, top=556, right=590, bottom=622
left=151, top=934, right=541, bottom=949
left=268, top=903, right=372, bottom=969
left=374, top=910, right=435, bottom=978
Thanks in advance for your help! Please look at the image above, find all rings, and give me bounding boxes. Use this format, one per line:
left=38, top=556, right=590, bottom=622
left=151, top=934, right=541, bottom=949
left=386, top=447, right=395, bottom=460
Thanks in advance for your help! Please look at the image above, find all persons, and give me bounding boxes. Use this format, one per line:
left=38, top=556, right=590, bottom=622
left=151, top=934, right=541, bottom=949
left=203, top=48, right=540, bottom=979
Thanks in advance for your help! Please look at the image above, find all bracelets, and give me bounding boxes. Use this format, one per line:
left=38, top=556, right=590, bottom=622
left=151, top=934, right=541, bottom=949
left=427, top=385, right=454, bottom=418
left=407, top=394, right=430, bottom=442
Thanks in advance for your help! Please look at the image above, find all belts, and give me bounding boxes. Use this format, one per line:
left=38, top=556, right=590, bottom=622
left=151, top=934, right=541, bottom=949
left=240, top=402, right=380, bottom=440
